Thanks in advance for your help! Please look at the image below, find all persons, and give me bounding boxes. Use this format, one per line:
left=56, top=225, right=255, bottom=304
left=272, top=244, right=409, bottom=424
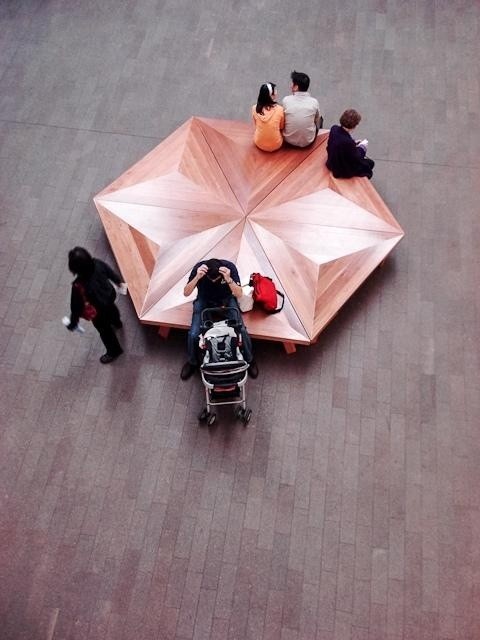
left=281, top=70, right=323, bottom=150
left=66, top=246, right=124, bottom=364
left=180, top=258, right=259, bottom=383
left=326, top=108, right=375, bottom=180
left=252, top=82, right=285, bottom=153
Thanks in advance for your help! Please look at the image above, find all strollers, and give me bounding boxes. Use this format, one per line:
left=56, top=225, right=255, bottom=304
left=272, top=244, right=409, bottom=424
left=194, top=306, right=256, bottom=429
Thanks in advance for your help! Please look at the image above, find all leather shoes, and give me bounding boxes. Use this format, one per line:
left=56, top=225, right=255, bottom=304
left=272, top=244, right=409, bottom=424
left=100, top=348, right=123, bottom=363
left=248, top=356, right=258, bottom=378
left=181, top=362, right=196, bottom=379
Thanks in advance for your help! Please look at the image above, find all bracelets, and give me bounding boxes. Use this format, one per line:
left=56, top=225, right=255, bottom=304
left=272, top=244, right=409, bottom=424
left=226, top=278, right=233, bottom=285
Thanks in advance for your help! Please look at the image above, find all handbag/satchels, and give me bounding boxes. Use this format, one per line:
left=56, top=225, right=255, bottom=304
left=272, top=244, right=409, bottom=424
left=73, top=282, right=97, bottom=321
left=249, top=273, right=284, bottom=314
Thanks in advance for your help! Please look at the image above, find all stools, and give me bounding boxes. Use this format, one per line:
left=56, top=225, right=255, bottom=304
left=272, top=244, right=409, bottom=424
left=93, top=115, right=408, bottom=356
